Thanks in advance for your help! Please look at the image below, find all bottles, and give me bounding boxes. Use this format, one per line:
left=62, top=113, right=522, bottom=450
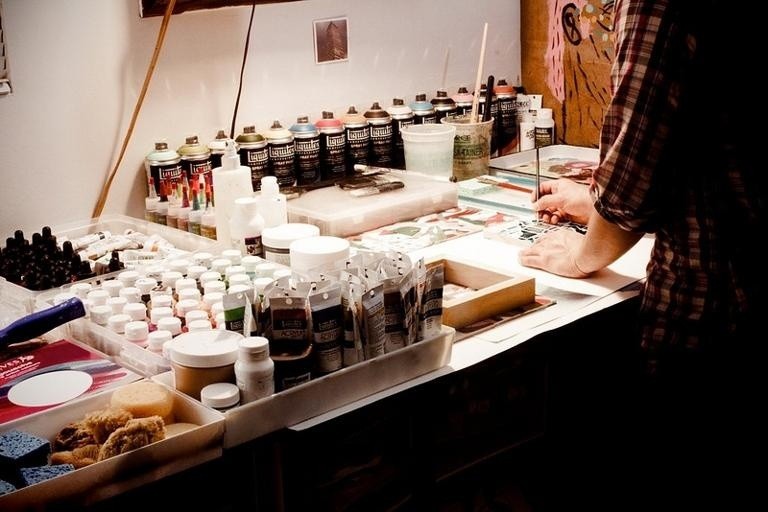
left=144, top=78, right=558, bottom=277
left=159, top=327, right=278, bottom=412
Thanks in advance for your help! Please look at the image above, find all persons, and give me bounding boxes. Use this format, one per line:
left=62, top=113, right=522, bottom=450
left=516, top=1, right=767, bottom=511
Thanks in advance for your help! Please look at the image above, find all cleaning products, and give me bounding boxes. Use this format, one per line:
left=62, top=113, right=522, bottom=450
left=212, top=140, right=288, bottom=254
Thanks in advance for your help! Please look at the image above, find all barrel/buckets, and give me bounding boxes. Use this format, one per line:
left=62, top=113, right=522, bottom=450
left=400, top=124, right=455, bottom=178
left=440, top=114, right=494, bottom=178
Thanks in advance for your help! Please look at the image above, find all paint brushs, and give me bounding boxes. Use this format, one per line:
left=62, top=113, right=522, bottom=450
left=535, top=145, right=540, bottom=226
left=472, top=23, right=488, bottom=124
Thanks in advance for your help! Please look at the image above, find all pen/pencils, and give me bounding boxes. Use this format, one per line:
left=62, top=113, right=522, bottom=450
left=485, top=76, right=494, bottom=120
